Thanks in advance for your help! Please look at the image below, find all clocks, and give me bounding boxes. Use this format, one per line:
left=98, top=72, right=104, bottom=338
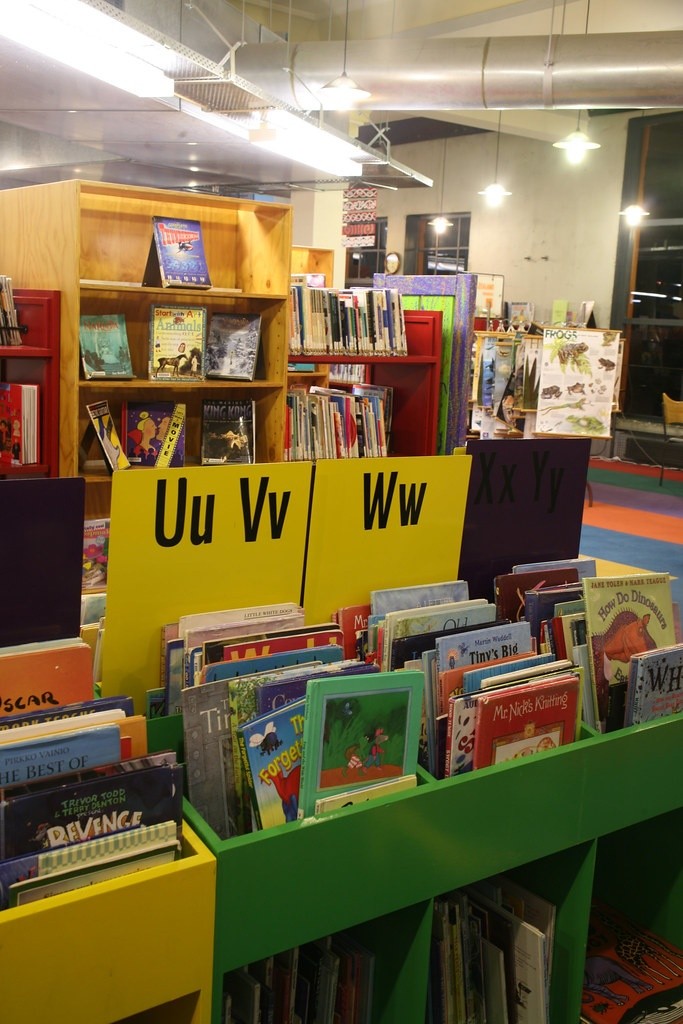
left=383, top=251, right=400, bottom=273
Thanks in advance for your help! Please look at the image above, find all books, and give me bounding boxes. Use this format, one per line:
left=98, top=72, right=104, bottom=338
left=148, top=304, right=207, bottom=382
left=0, top=275, right=23, bottom=346
left=200, top=399, right=255, bottom=466
left=0, top=381, right=40, bottom=466
left=290, top=273, right=407, bottom=356
left=86, top=399, right=131, bottom=472
left=207, top=311, right=262, bottom=382
left=153, top=216, right=212, bottom=291
left=468, top=300, right=625, bottom=439
left=0, top=558, right=683, bottom=1024
left=79, top=313, right=134, bottom=379
left=284, top=382, right=393, bottom=462
left=122, top=400, right=186, bottom=468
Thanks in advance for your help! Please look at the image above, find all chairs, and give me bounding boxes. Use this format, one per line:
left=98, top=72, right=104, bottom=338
left=659, top=391, right=683, bottom=486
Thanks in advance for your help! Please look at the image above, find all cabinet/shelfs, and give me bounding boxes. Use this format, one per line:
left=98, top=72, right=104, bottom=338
left=0, top=284, right=62, bottom=478
left=0, top=177, right=294, bottom=592
left=148, top=713, right=683, bottom=1024
left=286, top=302, right=443, bottom=456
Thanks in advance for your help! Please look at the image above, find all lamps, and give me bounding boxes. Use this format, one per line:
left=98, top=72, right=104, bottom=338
left=0, top=0, right=177, bottom=99
left=426, top=136, right=456, bottom=235
left=616, top=202, right=651, bottom=228
left=250, top=128, right=364, bottom=178
left=551, top=109, right=603, bottom=166
left=477, top=110, right=513, bottom=209
left=317, top=0, right=373, bottom=106
